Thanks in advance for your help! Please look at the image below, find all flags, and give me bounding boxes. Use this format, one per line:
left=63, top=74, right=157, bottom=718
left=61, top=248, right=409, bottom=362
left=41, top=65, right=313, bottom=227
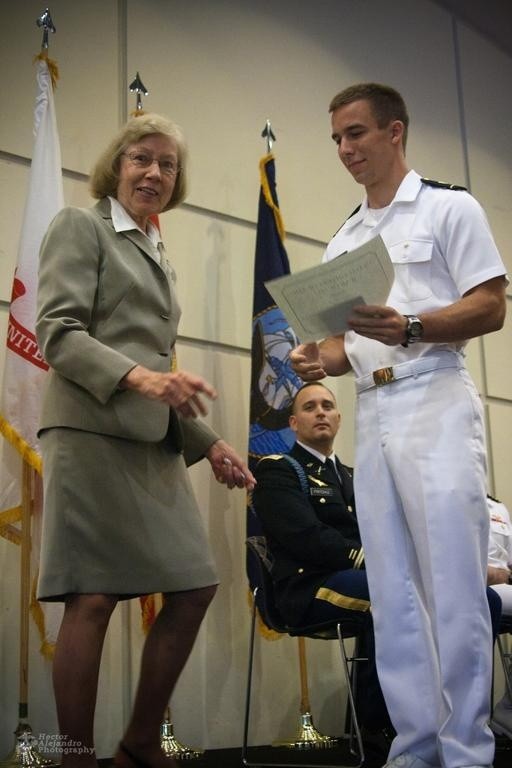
left=246, top=154, right=314, bottom=642
left=0, top=54, right=66, bottom=659
left=141, top=213, right=178, bottom=635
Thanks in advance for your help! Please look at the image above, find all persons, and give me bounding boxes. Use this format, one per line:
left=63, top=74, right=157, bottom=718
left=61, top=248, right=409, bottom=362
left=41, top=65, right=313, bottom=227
left=33, top=112, right=259, bottom=766
left=486, top=492, right=512, bottom=649
left=253, top=382, right=393, bottom=752
left=287, top=82, right=512, bottom=766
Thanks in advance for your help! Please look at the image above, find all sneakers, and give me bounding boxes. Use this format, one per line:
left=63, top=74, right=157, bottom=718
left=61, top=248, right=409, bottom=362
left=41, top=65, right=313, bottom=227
left=381, top=748, right=494, bottom=767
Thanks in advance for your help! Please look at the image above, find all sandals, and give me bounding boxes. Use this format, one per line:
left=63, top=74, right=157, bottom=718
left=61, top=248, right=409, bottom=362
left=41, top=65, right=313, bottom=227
left=110, top=739, right=179, bottom=767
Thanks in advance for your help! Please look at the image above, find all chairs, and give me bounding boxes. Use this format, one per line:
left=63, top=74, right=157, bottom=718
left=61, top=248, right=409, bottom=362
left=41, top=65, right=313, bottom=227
left=242, top=541, right=373, bottom=768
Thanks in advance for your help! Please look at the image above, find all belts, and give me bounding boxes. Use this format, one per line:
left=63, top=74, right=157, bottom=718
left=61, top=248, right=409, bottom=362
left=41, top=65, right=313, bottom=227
left=353, top=352, right=464, bottom=397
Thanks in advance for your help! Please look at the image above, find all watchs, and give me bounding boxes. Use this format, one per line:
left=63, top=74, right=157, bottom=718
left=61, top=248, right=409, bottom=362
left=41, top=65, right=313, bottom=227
left=401, top=315, right=425, bottom=352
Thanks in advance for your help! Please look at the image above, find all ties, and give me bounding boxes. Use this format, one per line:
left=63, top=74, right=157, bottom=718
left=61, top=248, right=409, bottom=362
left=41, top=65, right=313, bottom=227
left=325, top=455, right=340, bottom=485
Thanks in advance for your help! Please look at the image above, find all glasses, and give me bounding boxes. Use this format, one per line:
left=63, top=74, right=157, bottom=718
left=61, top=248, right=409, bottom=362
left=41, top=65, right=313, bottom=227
left=121, top=148, right=182, bottom=177
